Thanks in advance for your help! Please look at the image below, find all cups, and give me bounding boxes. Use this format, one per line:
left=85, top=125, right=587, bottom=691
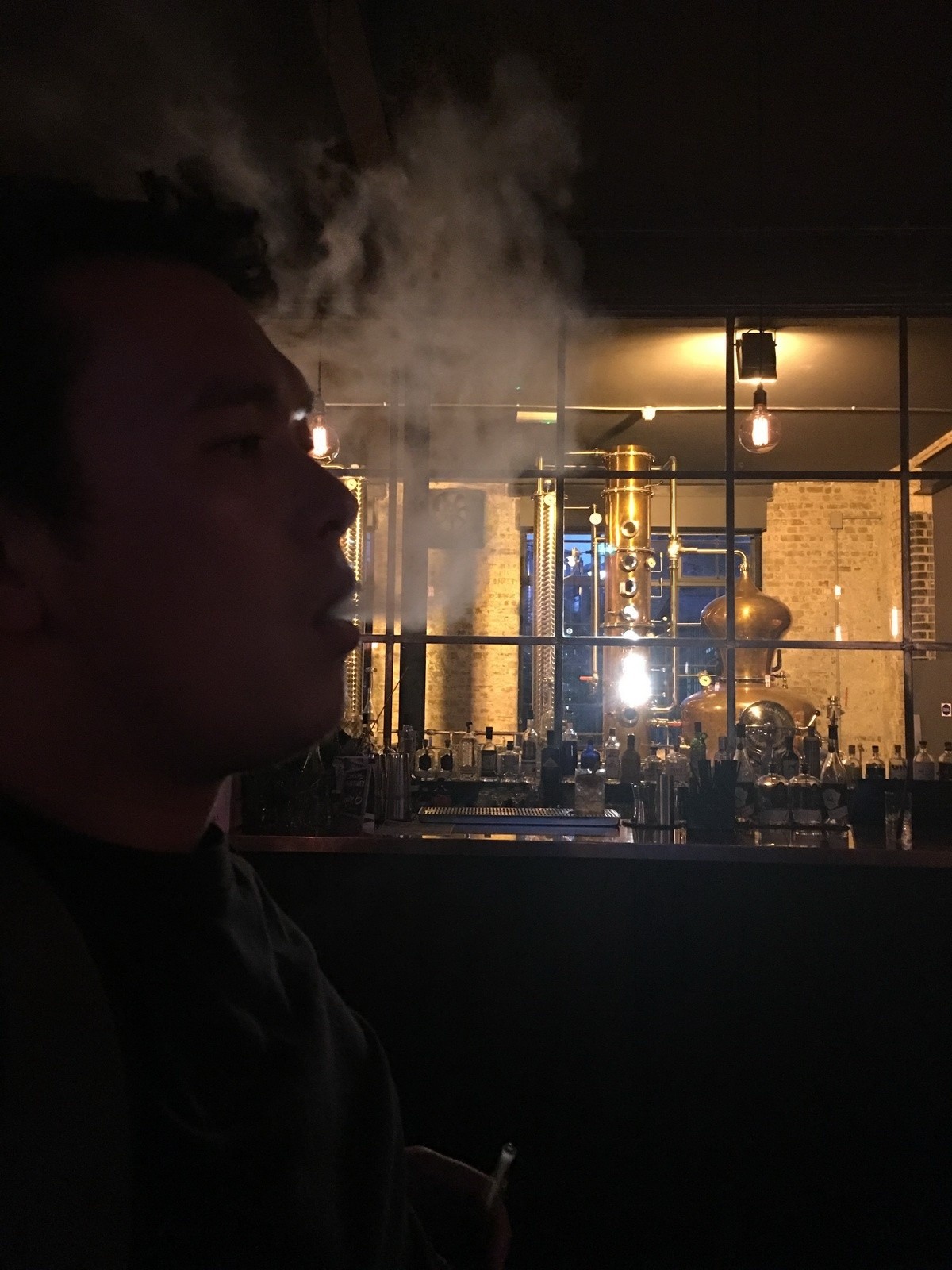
left=884, top=792, right=912, bottom=847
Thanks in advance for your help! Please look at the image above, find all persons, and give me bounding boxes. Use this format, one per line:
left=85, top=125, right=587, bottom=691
left=0, top=151, right=512, bottom=1268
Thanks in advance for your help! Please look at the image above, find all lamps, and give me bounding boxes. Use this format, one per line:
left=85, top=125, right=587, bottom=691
left=301, top=302, right=339, bottom=466
left=738, top=205, right=784, bottom=453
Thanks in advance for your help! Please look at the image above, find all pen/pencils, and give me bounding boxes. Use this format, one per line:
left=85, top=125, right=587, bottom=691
left=484, top=1142, right=518, bottom=1209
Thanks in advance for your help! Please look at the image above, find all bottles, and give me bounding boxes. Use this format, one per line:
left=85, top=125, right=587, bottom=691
left=503, top=741, right=519, bottom=777
left=540, top=730, right=560, bottom=782
left=790, top=764, right=822, bottom=829
left=666, top=737, right=687, bottom=780
left=622, top=734, right=640, bottom=783
left=779, top=736, right=799, bottom=779
left=522, top=710, right=538, bottom=770
left=645, top=747, right=664, bottom=781
left=581, top=740, right=600, bottom=773
left=821, top=723, right=847, bottom=783
left=755, top=765, right=789, bottom=826
left=562, top=710, right=578, bottom=778
left=760, top=736, right=779, bottom=775
left=603, top=728, right=622, bottom=784
left=439, top=738, right=457, bottom=773
left=479, top=727, right=497, bottom=778
left=691, top=722, right=706, bottom=769
left=459, top=721, right=477, bottom=775
left=713, top=737, right=731, bottom=761
left=415, top=739, right=434, bottom=777
left=844, top=741, right=952, bottom=780
left=731, top=723, right=753, bottom=782
left=803, top=727, right=820, bottom=779
